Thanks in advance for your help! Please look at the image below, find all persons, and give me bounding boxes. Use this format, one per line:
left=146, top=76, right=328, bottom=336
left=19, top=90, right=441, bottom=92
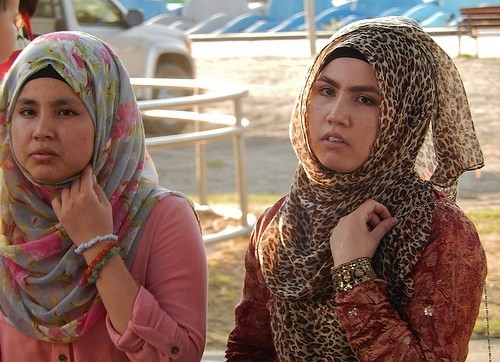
left=0, top=0, right=19, bottom=64
left=1, top=30, right=208, bottom=362
left=225, top=15, right=487, bottom=362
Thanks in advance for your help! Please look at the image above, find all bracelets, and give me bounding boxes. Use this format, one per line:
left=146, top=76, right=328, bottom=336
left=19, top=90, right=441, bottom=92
left=332, top=256, right=376, bottom=293
left=81, top=242, right=122, bottom=287
left=74, top=232, right=119, bottom=255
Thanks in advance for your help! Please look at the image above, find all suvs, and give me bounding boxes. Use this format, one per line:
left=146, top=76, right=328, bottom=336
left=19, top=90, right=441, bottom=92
left=21, top=0, right=196, bottom=137
